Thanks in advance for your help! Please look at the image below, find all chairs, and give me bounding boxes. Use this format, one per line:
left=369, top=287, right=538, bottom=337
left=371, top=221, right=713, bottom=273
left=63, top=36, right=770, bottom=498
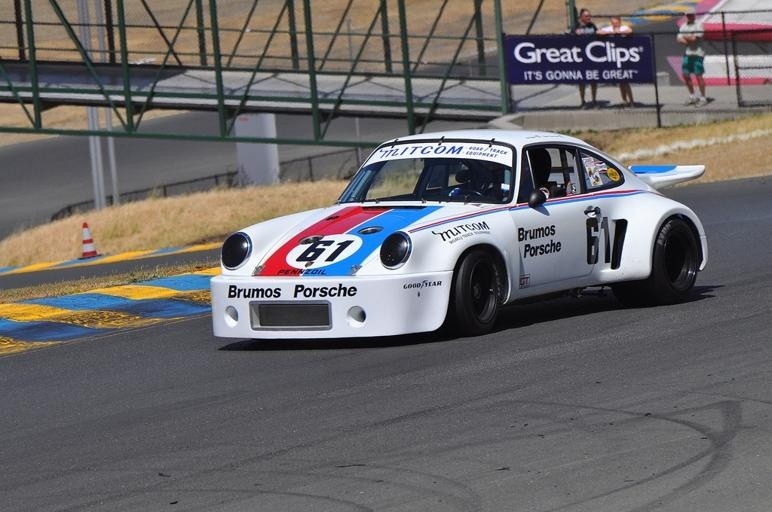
left=449, top=147, right=557, bottom=197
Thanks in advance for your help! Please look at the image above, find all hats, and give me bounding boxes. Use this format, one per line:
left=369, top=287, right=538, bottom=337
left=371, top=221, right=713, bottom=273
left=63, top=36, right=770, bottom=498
left=685, top=9, right=696, bottom=16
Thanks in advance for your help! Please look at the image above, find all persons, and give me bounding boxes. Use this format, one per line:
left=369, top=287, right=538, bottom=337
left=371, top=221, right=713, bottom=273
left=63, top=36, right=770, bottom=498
left=563, top=8, right=602, bottom=111
left=595, top=16, right=635, bottom=109
left=677, top=8, right=708, bottom=108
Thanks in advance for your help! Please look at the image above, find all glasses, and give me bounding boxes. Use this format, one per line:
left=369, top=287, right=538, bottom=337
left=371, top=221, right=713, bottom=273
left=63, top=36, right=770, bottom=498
left=585, top=14, right=591, bottom=18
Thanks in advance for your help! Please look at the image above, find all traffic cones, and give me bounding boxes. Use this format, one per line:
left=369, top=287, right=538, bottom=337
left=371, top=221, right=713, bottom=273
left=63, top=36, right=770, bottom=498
left=78, top=222, right=102, bottom=260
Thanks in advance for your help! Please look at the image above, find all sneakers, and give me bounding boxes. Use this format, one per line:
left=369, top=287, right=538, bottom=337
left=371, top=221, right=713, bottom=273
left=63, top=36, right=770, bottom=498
left=683, top=96, right=708, bottom=106
left=581, top=100, right=597, bottom=111
left=621, top=103, right=637, bottom=108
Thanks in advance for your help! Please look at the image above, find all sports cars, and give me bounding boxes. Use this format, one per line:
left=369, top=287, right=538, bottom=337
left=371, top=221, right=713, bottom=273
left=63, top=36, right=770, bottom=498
left=209, top=129, right=709, bottom=341
